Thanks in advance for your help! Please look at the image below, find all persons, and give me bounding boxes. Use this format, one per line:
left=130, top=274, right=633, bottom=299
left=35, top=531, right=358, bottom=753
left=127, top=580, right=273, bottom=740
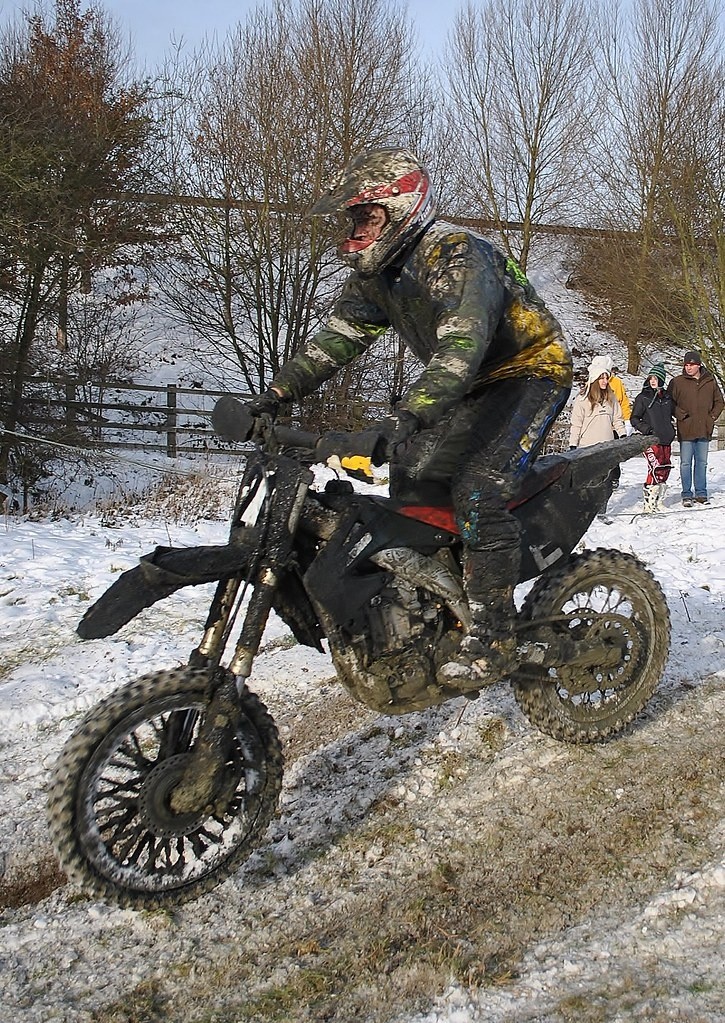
left=629, top=362, right=675, bottom=515
left=244, top=148, right=574, bottom=688
left=667, top=352, right=725, bottom=507
left=569, top=356, right=630, bottom=525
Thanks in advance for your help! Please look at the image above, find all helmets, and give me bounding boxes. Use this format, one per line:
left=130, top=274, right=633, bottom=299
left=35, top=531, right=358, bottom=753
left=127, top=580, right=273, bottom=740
left=310, top=147, right=437, bottom=276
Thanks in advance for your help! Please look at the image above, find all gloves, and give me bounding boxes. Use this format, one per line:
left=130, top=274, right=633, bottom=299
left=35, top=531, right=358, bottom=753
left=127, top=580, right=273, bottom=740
left=621, top=435, right=626, bottom=438
left=243, top=389, right=292, bottom=423
left=570, top=446, right=576, bottom=451
left=365, top=410, right=421, bottom=464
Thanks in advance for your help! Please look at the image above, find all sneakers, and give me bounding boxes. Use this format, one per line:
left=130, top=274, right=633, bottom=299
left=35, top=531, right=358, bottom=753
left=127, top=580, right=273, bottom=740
left=683, top=498, right=692, bottom=507
left=694, top=496, right=711, bottom=504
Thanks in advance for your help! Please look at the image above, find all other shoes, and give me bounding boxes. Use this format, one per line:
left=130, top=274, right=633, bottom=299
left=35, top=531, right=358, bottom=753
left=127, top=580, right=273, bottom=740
left=597, top=514, right=613, bottom=525
left=611, top=478, right=619, bottom=490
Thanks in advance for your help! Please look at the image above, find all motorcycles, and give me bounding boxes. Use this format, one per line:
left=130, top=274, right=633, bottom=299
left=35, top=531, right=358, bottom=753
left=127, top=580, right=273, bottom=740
left=48, top=395, right=673, bottom=918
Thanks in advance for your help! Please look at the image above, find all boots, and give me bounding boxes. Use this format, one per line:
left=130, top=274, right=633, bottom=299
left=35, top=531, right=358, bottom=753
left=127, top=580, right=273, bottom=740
left=436, top=546, right=524, bottom=688
left=655, top=484, right=674, bottom=513
left=642, top=483, right=671, bottom=519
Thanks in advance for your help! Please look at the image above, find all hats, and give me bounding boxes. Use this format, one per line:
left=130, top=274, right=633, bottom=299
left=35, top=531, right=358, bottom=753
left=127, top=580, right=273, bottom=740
left=644, top=362, right=666, bottom=389
left=580, top=364, right=610, bottom=401
left=684, top=352, right=701, bottom=366
left=592, top=356, right=614, bottom=372
